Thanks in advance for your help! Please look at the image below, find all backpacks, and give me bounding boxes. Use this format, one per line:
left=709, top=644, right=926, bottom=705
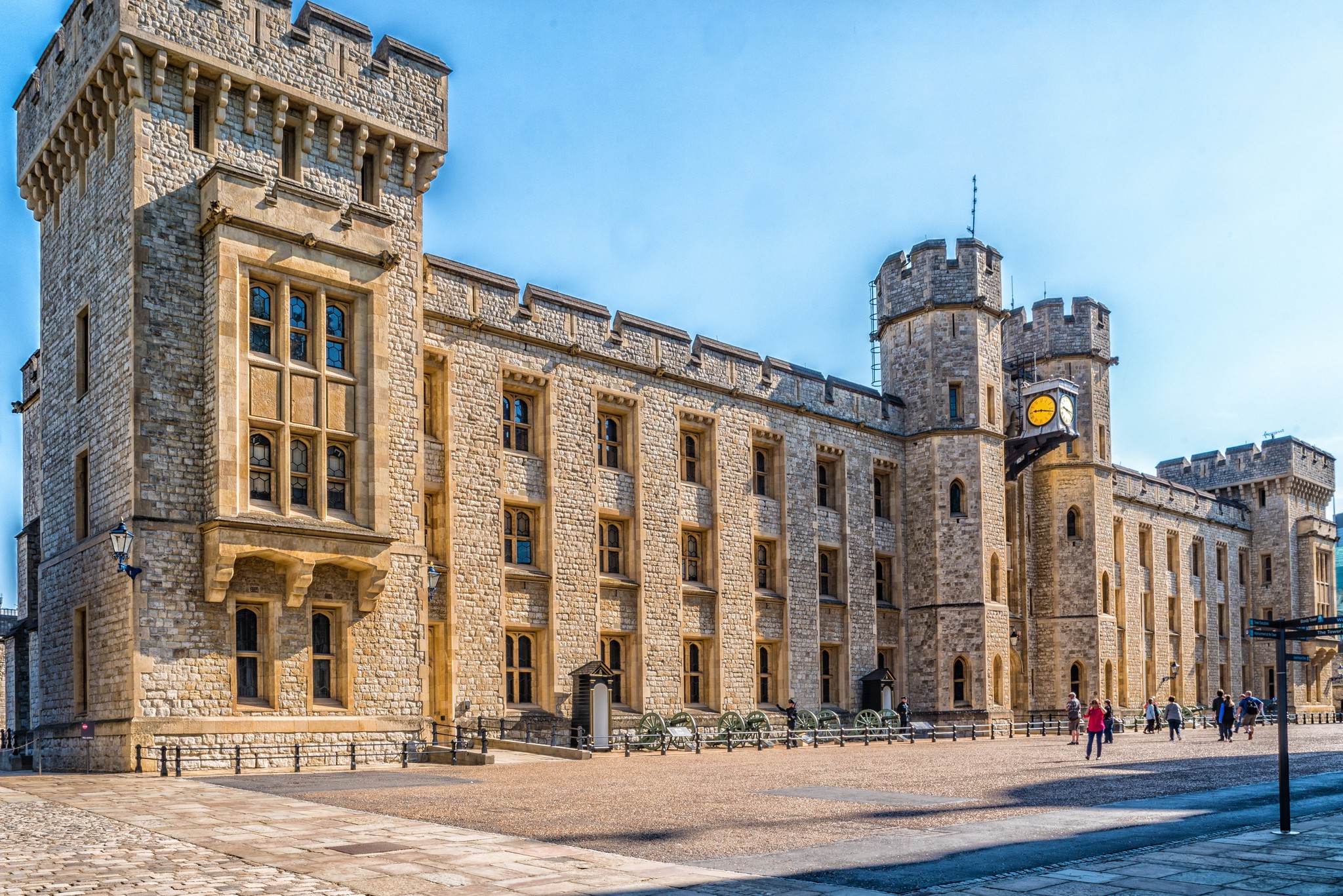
left=1242, top=697, right=1259, bottom=715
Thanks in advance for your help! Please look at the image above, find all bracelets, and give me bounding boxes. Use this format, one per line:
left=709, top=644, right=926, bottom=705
left=1100, top=706, right=1103, bottom=709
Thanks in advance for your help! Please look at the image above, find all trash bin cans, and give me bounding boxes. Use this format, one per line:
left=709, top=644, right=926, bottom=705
left=1069, top=719, right=1084, bottom=735
left=1112, top=719, right=1123, bottom=733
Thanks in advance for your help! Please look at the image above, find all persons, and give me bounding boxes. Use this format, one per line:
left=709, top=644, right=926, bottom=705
left=1232, top=691, right=1263, bottom=740
left=1212, top=688, right=1235, bottom=742
left=1142, top=696, right=1161, bottom=734
left=1085, top=698, right=1107, bottom=760
left=1162, top=695, right=1183, bottom=741
left=776, top=699, right=799, bottom=749
left=1065, top=692, right=1081, bottom=745
left=896, top=696, right=910, bottom=736
left=1102, top=699, right=1114, bottom=744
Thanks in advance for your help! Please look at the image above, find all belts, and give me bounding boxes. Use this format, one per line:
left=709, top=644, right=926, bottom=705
left=901, top=712, right=908, bottom=714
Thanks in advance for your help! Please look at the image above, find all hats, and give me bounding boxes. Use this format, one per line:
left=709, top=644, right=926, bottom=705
left=788, top=699, right=795, bottom=704
left=901, top=696, right=907, bottom=699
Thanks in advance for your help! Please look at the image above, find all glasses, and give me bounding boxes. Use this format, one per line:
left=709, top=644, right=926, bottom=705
left=1239, top=696, right=1242, bottom=698
left=1092, top=702, right=1098, bottom=705
left=1068, top=695, right=1073, bottom=696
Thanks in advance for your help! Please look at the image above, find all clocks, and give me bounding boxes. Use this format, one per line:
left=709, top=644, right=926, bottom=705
left=1060, top=395, right=1074, bottom=424
left=1028, top=396, right=1055, bottom=426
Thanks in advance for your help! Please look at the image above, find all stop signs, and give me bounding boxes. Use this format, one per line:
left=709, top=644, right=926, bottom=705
left=82, top=725, right=87, bottom=729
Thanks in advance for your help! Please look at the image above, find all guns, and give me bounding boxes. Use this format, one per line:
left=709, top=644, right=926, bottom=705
left=788, top=701, right=798, bottom=720
left=906, top=700, right=912, bottom=719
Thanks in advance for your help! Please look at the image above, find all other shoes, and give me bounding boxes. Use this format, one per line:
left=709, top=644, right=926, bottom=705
left=1224, top=739, right=1228, bottom=742
left=1086, top=756, right=1090, bottom=760
left=1243, top=730, right=1250, bottom=734
left=1067, top=742, right=1075, bottom=745
left=1233, top=730, right=1238, bottom=733
left=1169, top=739, right=1175, bottom=741
left=1218, top=738, right=1224, bottom=741
left=1148, top=732, right=1152, bottom=734
left=791, top=744, right=798, bottom=748
left=1102, top=742, right=1109, bottom=744
left=1143, top=731, right=1148, bottom=734
left=1109, top=741, right=1113, bottom=744
left=1075, top=742, right=1079, bottom=744
left=784, top=742, right=787, bottom=744
left=1158, top=731, right=1160, bottom=734
left=1177, top=734, right=1182, bottom=740
left=1248, top=730, right=1253, bottom=740
left=1152, top=732, right=1154, bottom=734
left=1229, top=737, right=1233, bottom=742
left=1096, top=757, right=1101, bottom=759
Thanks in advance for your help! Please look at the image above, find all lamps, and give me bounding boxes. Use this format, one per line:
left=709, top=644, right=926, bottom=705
left=109, top=516, right=143, bottom=580
left=427, top=561, right=441, bottom=602
left=1162, top=659, right=1180, bottom=682
left=1010, top=625, right=1019, bottom=646
left=1331, top=663, right=1343, bottom=681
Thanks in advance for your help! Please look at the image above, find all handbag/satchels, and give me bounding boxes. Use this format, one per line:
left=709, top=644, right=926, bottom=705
left=1143, top=713, right=1146, bottom=717
left=1068, top=711, right=1079, bottom=721
